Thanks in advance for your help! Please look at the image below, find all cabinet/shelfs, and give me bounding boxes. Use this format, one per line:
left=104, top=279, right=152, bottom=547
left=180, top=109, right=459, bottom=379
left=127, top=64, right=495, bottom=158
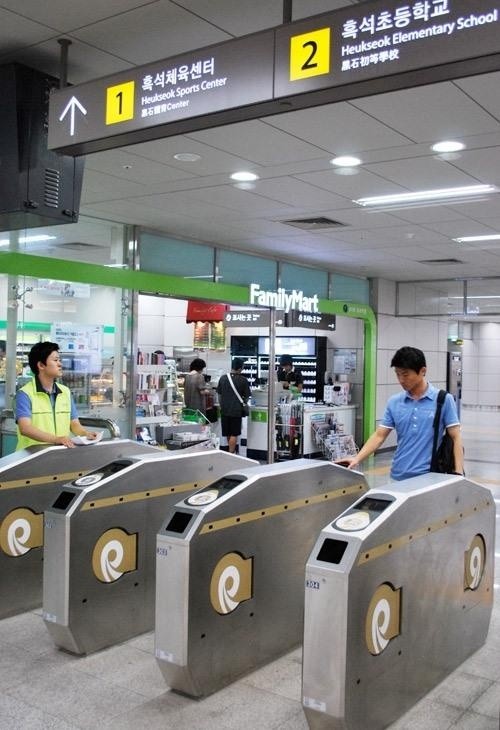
left=230, top=334, right=327, bottom=402
left=120, top=344, right=174, bottom=446
left=0, top=320, right=52, bottom=365
left=58, top=370, right=113, bottom=411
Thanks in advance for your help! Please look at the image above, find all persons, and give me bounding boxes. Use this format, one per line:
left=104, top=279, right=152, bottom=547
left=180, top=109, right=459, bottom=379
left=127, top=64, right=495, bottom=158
left=277, top=354, right=303, bottom=393
left=217, top=358, right=252, bottom=455
left=14, top=341, right=97, bottom=453
left=0, top=340, right=23, bottom=382
left=334, top=346, right=464, bottom=481
left=183, top=358, right=210, bottom=416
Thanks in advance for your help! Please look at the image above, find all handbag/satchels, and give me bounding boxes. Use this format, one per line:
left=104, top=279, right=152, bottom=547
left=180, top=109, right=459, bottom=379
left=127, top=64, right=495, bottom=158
left=429, top=388, right=465, bottom=475
left=241, top=403, right=250, bottom=418
